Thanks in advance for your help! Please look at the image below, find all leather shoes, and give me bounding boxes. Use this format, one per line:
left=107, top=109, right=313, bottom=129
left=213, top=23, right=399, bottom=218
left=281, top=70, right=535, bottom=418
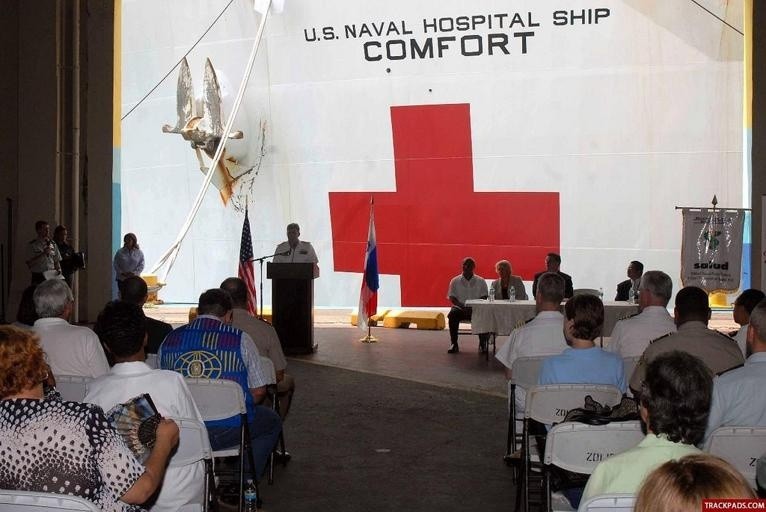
left=447, top=342, right=459, bottom=354
left=479, top=343, right=487, bottom=354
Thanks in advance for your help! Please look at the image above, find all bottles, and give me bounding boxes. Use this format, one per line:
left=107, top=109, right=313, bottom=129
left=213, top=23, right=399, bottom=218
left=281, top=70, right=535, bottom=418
left=244, top=478, right=256, bottom=512
left=629, top=288, right=635, bottom=305
left=510, top=285, right=516, bottom=302
left=599, top=288, right=604, bottom=303
left=489, top=284, right=496, bottom=302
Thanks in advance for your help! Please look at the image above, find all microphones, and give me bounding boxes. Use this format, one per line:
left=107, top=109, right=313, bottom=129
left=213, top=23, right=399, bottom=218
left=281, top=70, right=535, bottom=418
left=280, top=251, right=291, bottom=256
left=46, top=239, right=50, bottom=257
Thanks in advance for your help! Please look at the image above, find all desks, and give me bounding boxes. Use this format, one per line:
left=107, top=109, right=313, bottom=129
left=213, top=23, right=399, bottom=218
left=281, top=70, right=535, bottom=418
left=459, top=295, right=646, bottom=369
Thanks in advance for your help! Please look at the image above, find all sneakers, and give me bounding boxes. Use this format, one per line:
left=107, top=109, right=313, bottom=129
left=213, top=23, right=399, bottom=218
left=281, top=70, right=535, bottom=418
left=267, top=448, right=292, bottom=465
left=219, top=489, right=263, bottom=512
left=502, top=448, right=523, bottom=462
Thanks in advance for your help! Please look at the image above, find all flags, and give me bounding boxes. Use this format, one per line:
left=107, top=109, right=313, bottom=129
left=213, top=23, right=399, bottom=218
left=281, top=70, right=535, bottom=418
left=358, top=205, right=380, bottom=332
left=237, top=209, right=257, bottom=319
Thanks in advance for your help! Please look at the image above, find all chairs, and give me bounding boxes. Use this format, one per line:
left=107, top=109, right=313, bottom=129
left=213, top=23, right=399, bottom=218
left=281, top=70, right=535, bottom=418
left=457, top=318, right=497, bottom=362
left=572, top=286, right=606, bottom=349
left=0, top=352, right=295, bottom=510
left=503, top=354, right=766, bottom=512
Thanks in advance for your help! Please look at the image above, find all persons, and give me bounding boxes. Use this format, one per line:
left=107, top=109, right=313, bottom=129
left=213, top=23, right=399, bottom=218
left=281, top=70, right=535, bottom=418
left=531, top=253, right=575, bottom=302
left=273, top=222, right=319, bottom=264
left=53, top=224, right=76, bottom=288
left=23, top=220, right=62, bottom=283
left=113, top=232, right=144, bottom=290
left=488, top=258, right=529, bottom=301
left=616, top=261, right=644, bottom=301
left=445, top=255, right=488, bottom=353
